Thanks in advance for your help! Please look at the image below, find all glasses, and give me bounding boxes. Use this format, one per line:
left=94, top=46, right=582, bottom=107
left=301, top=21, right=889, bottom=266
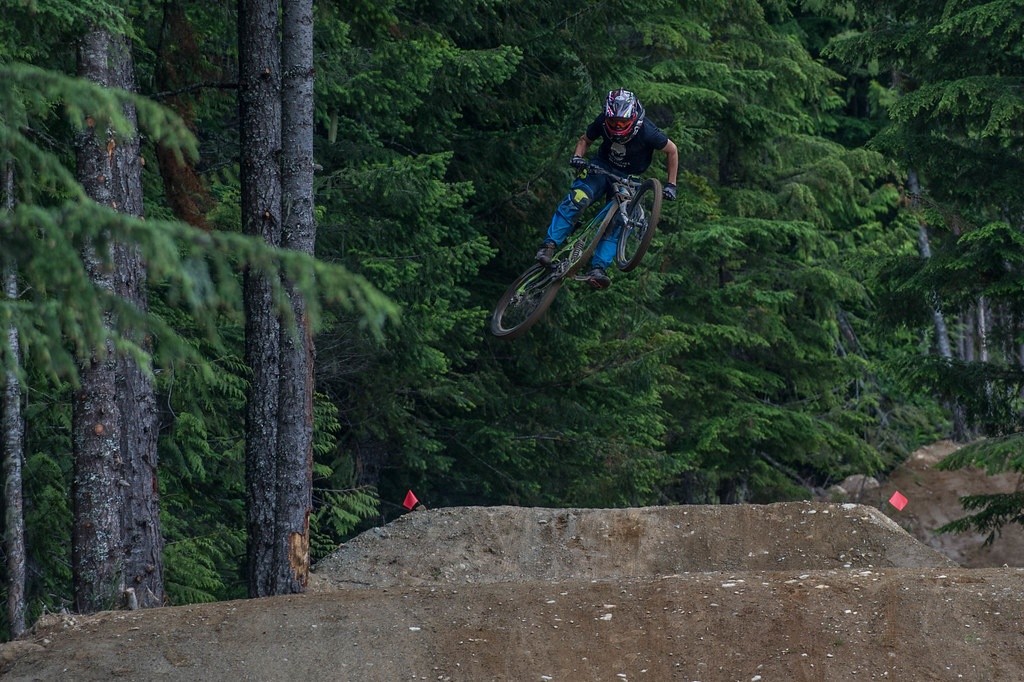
left=607, top=117, right=632, bottom=128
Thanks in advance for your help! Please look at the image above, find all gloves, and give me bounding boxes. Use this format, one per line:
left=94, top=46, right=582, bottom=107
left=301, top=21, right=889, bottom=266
left=570, top=155, right=588, bottom=174
left=662, top=182, right=676, bottom=201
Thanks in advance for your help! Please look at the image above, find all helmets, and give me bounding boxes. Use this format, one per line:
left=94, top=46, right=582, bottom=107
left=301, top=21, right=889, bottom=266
left=603, top=87, right=645, bottom=144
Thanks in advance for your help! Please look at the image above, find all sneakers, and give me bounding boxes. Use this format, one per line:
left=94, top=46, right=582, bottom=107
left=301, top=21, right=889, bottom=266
left=535, top=240, right=557, bottom=267
left=586, top=267, right=611, bottom=290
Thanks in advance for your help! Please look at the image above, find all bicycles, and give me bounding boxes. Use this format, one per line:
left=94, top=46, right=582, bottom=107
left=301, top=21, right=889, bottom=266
left=489, top=158, right=673, bottom=343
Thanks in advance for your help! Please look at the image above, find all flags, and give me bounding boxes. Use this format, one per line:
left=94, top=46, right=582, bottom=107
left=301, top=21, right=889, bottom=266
left=889, top=490, right=908, bottom=511
left=403, top=490, right=419, bottom=509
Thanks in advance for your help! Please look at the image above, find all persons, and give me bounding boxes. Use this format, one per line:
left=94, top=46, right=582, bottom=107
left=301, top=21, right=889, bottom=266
left=535, top=85, right=679, bottom=289
